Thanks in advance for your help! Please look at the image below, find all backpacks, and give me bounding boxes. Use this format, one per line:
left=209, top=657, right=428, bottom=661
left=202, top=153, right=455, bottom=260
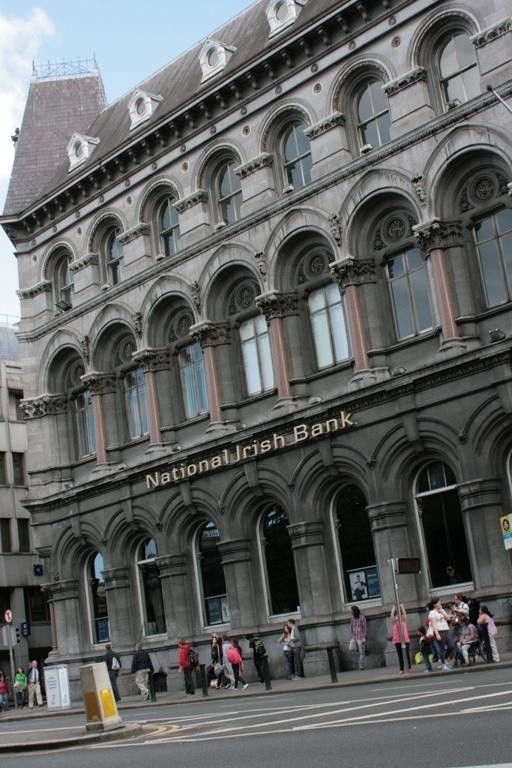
left=186, top=648, right=200, bottom=669
left=110, top=656, right=120, bottom=671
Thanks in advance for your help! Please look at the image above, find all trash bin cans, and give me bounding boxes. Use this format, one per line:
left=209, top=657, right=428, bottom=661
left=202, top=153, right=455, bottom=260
left=152, top=672, right=168, bottom=692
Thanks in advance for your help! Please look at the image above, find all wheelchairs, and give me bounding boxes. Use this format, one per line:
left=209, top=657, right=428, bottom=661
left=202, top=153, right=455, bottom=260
left=456, top=620, right=492, bottom=668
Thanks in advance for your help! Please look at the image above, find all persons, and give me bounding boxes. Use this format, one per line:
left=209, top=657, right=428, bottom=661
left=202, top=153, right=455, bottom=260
left=353, top=574, right=366, bottom=598
left=177, top=637, right=198, bottom=697
left=103, top=643, right=122, bottom=703
left=0, top=659, right=45, bottom=712
left=130, top=642, right=154, bottom=700
left=348, top=604, right=369, bottom=670
left=205, top=618, right=304, bottom=691
left=390, top=591, right=501, bottom=672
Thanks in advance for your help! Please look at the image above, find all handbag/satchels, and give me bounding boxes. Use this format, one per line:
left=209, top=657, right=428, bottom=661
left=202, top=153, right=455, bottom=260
left=213, top=663, right=224, bottom=677
left=228, top=646, right=240, bottom=665
left=415, top=652, right=425, bottom=664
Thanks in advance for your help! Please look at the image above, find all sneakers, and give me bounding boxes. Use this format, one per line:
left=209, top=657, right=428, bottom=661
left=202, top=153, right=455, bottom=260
left=114, top=679, right=267, bottom=706
left=0, top=702, right=46, bottom=713
left=285, top=655, right=501, bottom=681
